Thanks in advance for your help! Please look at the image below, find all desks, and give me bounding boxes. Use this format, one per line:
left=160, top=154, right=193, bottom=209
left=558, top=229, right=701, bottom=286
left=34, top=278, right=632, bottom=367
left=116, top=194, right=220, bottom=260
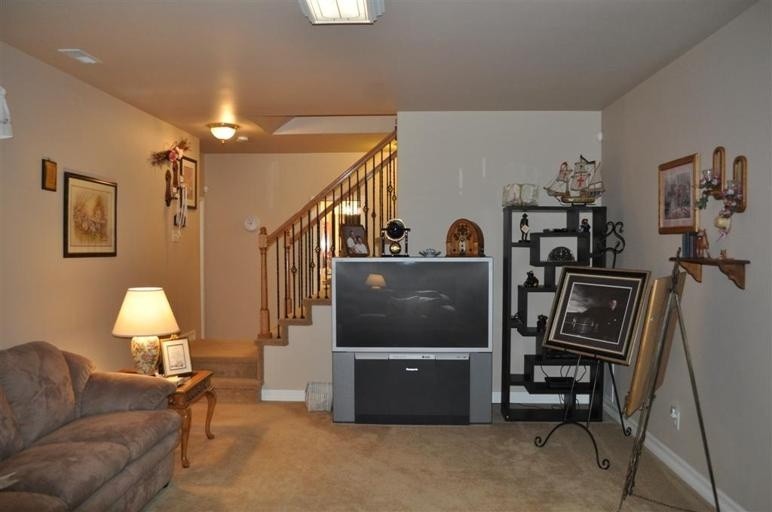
left=115, top=367, right=217, bottom=471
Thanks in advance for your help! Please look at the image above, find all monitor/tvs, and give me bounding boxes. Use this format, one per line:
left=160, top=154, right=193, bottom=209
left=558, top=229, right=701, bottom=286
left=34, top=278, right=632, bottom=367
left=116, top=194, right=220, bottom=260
left=331, top=256, right=493, bottom=352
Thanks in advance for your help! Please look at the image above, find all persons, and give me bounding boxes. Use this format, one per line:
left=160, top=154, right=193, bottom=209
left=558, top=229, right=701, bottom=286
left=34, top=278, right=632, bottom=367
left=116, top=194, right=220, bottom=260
left=601, top=298, right=622, bottom=329
left=347, top=231, right=368, bottom=254
left=520, top=213, right=529, bottom=240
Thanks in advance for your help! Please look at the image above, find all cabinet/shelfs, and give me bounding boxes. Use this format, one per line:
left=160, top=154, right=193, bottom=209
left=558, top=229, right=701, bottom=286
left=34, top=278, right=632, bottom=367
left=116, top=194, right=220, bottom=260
left=502, top=205, right=606, bottom=425
left=332, top=350, right=494, bottom=425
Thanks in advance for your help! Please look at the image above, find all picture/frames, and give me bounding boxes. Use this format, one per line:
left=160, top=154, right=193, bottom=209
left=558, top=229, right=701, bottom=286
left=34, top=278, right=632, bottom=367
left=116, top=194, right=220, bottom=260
left=158, top=336, right=193, bottom=377
left=656, top=154, right=699, bottom=233
left=339, top=222, right=371, bottom=258
left=61, top=171, right=118, bottom=258
left=541, top=266, right=651, bottom=367
left=180, top=155, right=197, bottom=210
left=40, top=158, right=57, bottom=192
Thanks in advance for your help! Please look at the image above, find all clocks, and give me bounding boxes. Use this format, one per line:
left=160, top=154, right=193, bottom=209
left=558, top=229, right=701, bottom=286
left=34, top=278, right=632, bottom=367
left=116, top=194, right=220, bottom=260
left=244, top=214, right=259, bottom=233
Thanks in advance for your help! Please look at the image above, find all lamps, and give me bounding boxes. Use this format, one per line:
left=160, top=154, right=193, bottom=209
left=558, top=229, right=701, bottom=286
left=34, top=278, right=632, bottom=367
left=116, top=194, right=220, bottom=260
left=110, top=285, right=182, bottom=376
left=206, top=121, right=238, bottom=140
left=300, top=0, right=386, bottom=26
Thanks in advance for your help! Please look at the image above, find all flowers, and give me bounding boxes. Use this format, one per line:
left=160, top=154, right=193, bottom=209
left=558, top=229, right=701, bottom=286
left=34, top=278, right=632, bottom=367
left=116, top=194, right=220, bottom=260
left=150, top=137, right=190, bottom=165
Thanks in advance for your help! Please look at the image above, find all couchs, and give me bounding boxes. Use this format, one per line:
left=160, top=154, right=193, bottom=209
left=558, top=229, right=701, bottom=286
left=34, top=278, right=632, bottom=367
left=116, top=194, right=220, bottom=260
left=0, top=342, right=182, bottom=510
